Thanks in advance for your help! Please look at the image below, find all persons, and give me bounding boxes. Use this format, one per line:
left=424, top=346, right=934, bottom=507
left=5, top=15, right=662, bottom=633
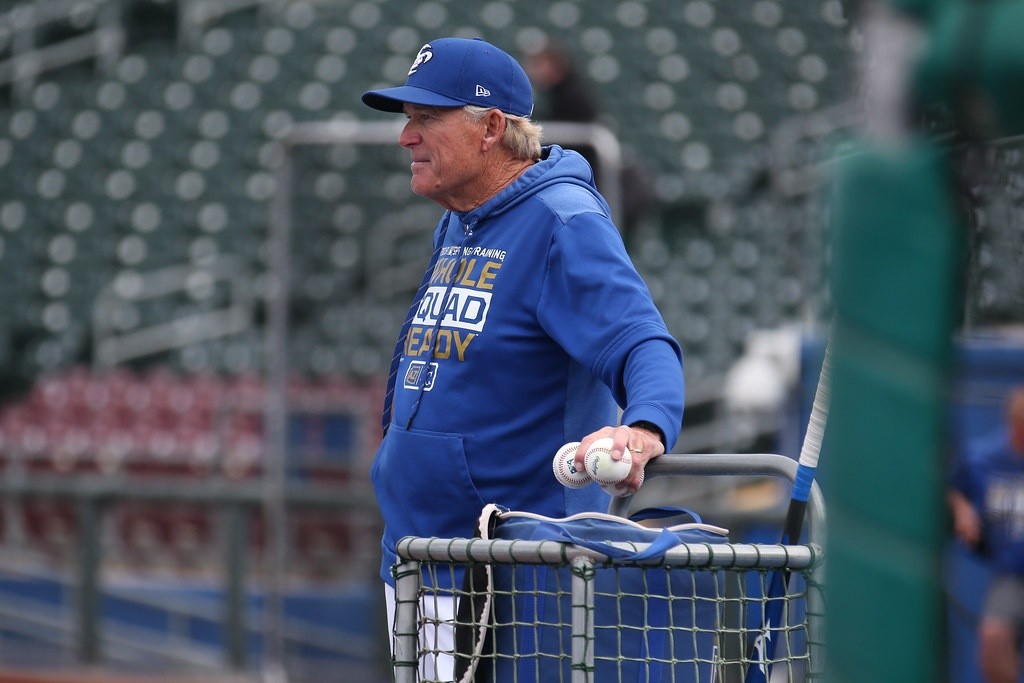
left=362, top=38, right=686, bottom=683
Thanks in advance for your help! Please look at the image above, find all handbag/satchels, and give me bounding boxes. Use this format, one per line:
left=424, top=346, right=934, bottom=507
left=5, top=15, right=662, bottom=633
left=451, top=503, right=730, bottom=683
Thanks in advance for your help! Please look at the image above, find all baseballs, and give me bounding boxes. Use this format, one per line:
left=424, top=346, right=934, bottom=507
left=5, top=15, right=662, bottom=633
left=552, top=441, right=595, bottom=489
left=584, top=437, right=634, bottom=486
left=600, top=466, right=646, bottom=498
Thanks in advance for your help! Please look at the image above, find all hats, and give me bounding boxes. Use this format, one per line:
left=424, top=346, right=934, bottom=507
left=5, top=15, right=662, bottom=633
left=362, top=37, right=535, bottom=119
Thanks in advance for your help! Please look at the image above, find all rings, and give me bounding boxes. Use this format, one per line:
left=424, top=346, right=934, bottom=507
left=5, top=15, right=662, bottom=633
left=630, top=447, right=643, bottom=455
